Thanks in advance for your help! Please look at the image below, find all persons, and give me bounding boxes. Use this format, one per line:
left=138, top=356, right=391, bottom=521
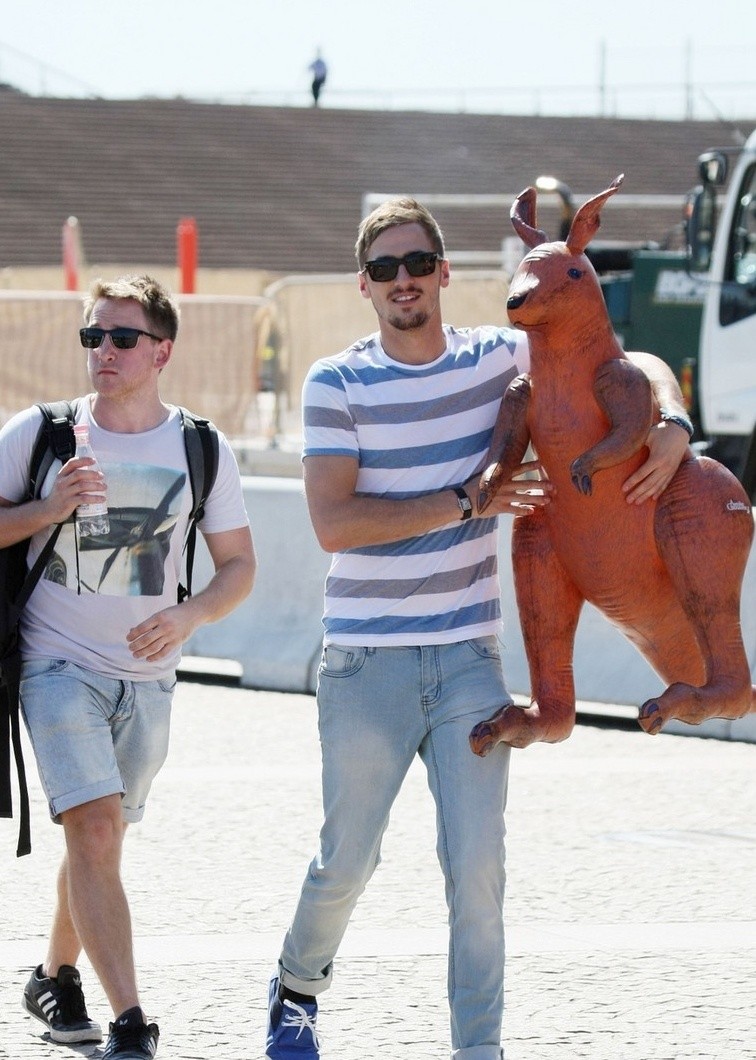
left=309, top=48, right=326, bottom=107
left=0, top=280, right=257, bottom=1060
left=261, top=192, right=695, bottom=1060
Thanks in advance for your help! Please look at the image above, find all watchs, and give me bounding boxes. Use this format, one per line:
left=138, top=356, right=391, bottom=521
left=451, top=487, right=473, bottom=521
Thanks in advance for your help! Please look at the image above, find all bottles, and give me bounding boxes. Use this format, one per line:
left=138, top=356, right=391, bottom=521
left=74, top=424, right=110, bottom=538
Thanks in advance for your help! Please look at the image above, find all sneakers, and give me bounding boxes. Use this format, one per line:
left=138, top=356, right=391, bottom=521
left=20, top=963, right=101, bottom=1043
left=101, top=1006, right=160, bottom=1060
left=266, top=977, right=320, bottom=1059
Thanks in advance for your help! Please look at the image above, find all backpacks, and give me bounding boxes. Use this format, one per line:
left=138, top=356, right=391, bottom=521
left=2, top=400, right=220, bottom=690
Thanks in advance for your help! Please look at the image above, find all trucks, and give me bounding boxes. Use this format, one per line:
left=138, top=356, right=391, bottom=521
left=625, top=125, right=755, bottom=508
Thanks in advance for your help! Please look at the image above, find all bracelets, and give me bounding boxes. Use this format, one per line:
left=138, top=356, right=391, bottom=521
left=659, top=409, right=695, bottom=437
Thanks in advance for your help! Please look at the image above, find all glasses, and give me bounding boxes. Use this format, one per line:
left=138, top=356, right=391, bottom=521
left=361, top=252, right=444, bottom=282
left=79, top=327, right=162, bottom=351
